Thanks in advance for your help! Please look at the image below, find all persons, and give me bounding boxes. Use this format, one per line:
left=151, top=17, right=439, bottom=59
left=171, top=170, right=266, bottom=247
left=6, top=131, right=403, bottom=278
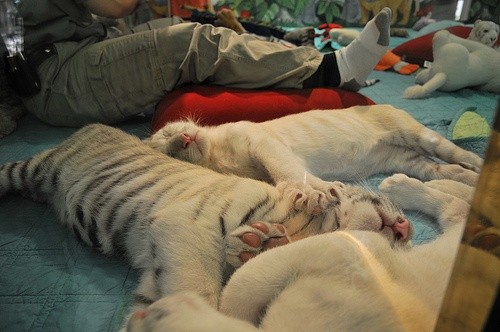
left=181, top=9, right=314, bottom=47
left=0, top=0, right=391, bottom=128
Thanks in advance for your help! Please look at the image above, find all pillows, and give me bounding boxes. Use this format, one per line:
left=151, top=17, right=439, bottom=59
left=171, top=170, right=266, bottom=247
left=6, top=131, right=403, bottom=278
left=158, top=80, right=375, bottom=140
